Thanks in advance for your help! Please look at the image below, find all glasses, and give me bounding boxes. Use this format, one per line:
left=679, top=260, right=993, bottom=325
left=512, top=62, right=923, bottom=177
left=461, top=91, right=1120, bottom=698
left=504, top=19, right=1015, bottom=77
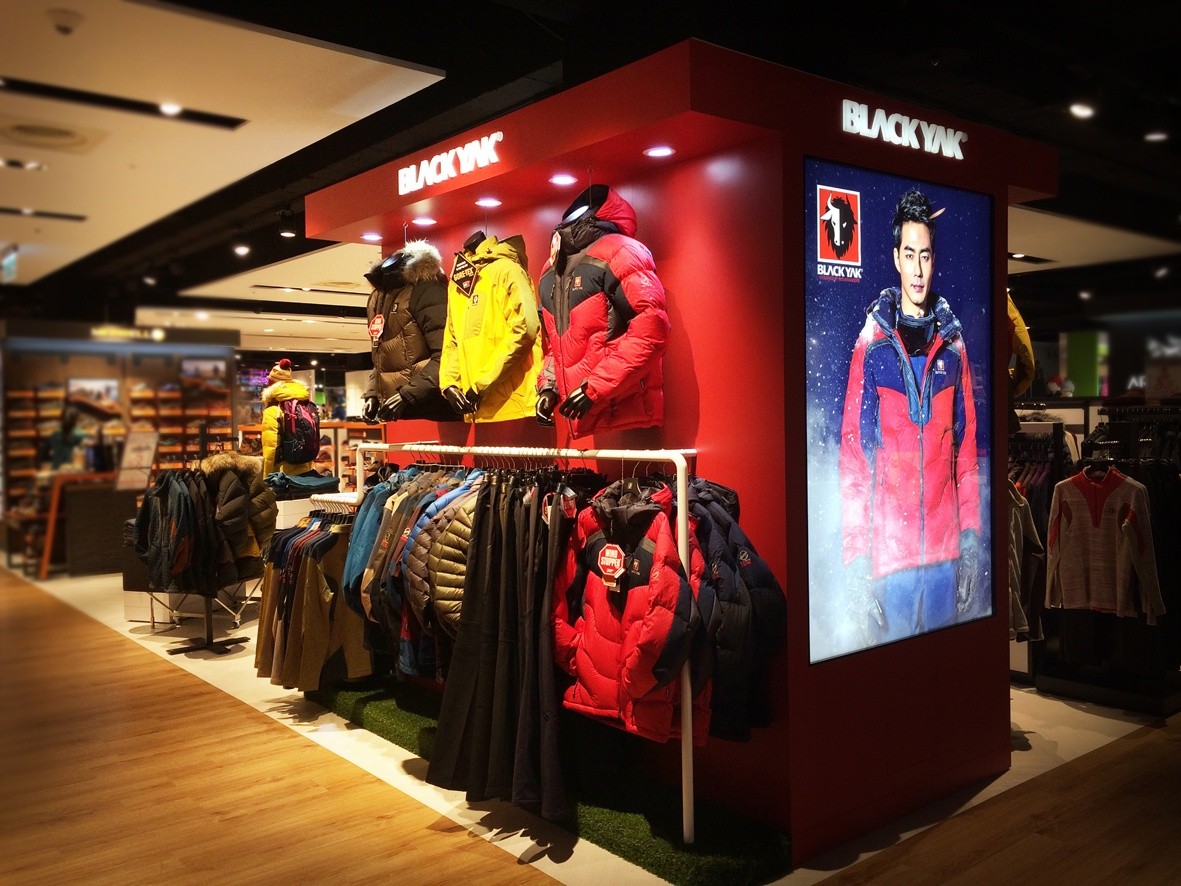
left=896, top=319, right=936, bottom=353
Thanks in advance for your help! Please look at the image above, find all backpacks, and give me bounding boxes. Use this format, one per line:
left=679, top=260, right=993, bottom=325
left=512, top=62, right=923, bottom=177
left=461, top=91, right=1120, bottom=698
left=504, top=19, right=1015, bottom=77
left=267, top=400, right=319, bottom=464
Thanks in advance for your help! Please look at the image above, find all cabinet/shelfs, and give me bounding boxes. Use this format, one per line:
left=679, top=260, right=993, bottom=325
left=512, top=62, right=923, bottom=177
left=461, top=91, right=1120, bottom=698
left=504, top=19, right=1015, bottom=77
left=4, top=388, right=232, bottom=499
left=238, top=420, right=386, bottom=489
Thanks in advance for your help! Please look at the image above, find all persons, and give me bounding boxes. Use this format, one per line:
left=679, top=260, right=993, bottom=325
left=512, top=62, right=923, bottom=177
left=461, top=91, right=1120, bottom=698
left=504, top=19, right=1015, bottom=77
left=47, top=406, right=90, bottom=472
left=361, top=184, right=672, bottom=440
left=842, top=190, right=981, bottom=648
left=261, top=358, right=313, bottom=481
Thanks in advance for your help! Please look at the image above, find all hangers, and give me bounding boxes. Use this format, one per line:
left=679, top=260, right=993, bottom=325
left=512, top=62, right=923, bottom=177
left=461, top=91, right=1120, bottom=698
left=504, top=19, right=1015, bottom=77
left=382, top=444, right=692, bottom=499
left=1007, top=401, right=1181, bottom=475
left=309, top=493, right=356, bottom=524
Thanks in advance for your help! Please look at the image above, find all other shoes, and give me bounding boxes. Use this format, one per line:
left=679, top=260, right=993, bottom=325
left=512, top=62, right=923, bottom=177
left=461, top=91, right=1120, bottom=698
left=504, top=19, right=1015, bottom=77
left=351, top=444, right=358, bottom=449
left=320, top=469, right=333, bottom=478
left=314, top=452, right=331, bottom=462
left=320, top=435, right=330, bottom=445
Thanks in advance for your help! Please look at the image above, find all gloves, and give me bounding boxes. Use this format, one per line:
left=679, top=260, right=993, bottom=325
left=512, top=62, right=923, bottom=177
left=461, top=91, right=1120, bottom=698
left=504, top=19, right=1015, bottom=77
left=846, top=556, right=884, bottom=646
left=957, top=528, right=980, bottom=616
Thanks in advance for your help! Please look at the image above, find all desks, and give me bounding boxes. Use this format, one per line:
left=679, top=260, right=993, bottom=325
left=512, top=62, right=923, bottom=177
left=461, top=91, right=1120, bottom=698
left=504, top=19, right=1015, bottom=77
left=122, top=546, right=247, bottom=623
left=1, top=510, right=46, bottom=576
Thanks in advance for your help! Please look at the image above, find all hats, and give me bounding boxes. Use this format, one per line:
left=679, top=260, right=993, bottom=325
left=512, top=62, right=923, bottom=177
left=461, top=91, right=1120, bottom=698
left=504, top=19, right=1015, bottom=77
left=1061, top=377, right=1074, bottom=392
left=268, top=359, right=292, bottom=386
left=1047, top=375, right=1061, bottom=386
